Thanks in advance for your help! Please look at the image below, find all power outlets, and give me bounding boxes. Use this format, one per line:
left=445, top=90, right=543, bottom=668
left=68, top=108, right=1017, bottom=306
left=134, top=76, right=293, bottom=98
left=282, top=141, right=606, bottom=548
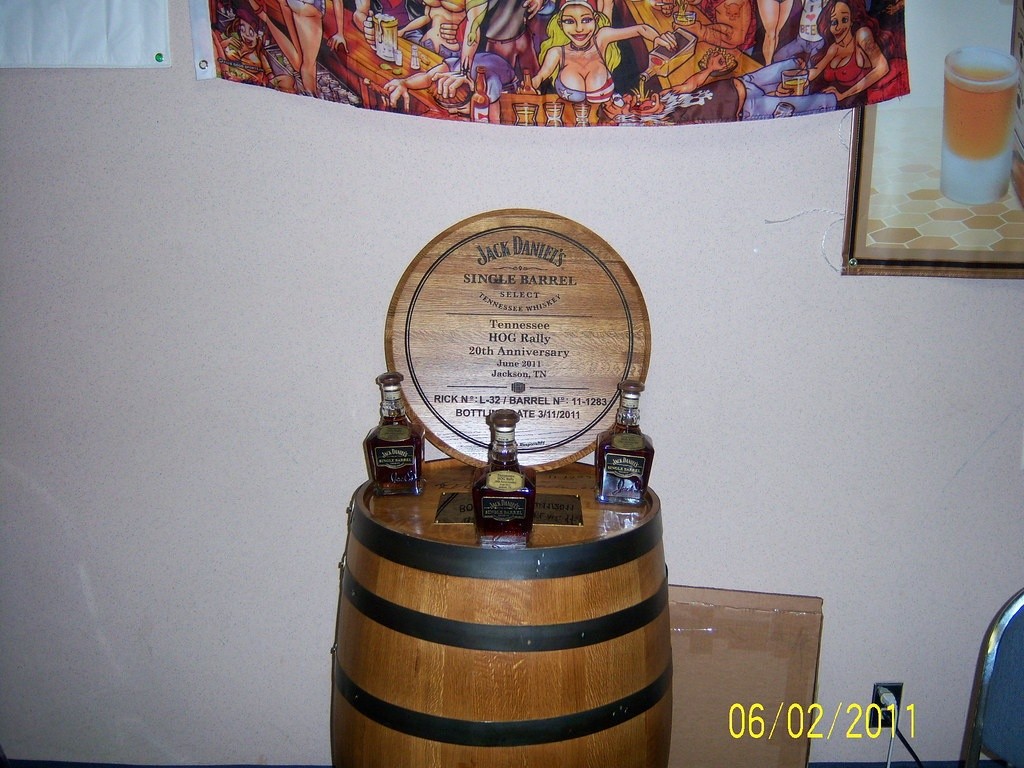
left=868, top=683, right=903, bottom=729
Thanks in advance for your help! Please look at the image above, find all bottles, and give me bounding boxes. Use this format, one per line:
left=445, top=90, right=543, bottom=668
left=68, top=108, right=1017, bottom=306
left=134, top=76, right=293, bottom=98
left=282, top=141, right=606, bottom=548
left=595, top=380, right=656, bottom=506
left=472, top=410, right=538, bottom=548
left=362, top=372, right=425, bottom=496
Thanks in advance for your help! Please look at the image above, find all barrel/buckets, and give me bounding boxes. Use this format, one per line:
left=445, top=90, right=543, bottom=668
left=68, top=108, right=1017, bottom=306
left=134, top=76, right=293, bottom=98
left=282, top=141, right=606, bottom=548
left=329, top=458, right=674, bottom=768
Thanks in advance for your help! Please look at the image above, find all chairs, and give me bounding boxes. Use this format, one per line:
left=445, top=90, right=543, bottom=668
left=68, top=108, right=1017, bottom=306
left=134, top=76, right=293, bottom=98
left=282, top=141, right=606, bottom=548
left=955, top=588, right=1024, bottom=768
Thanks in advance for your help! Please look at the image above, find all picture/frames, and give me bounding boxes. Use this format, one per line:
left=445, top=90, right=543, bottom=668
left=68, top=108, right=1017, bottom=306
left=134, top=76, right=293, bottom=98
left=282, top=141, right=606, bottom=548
left=840, top=0, right=1024, bottom=280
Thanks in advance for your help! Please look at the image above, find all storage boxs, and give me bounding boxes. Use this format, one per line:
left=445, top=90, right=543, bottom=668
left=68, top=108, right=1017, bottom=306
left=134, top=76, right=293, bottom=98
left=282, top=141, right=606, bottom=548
left=666, top=585, right=823, bottom=767
